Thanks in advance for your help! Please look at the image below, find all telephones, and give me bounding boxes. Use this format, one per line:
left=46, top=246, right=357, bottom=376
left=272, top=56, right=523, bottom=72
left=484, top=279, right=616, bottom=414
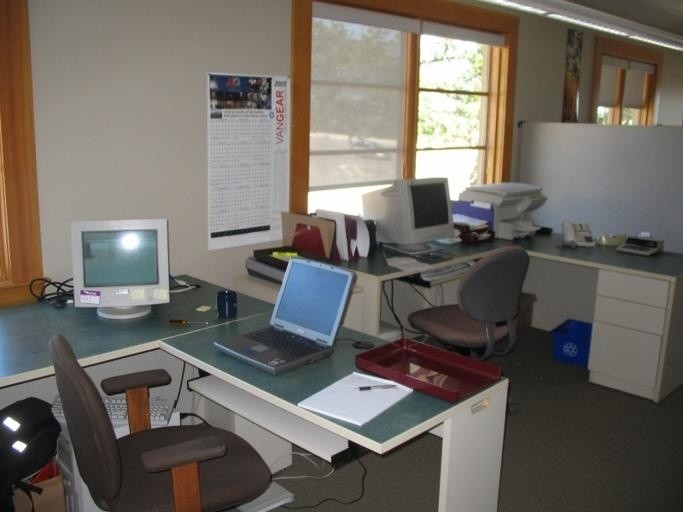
left=561, top=221, right=596, bottom=247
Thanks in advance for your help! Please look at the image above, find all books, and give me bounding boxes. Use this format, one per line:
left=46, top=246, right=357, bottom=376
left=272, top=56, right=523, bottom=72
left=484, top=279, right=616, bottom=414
left=299, top=373, right=413, bottom=428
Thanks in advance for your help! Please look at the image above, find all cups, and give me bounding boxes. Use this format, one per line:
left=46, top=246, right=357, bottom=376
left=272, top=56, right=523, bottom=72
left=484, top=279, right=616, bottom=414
left=216, top=288, right=239, bottom=319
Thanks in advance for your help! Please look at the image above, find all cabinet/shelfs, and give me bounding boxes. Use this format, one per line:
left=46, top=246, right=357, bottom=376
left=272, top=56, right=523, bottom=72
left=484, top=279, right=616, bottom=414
left=586, top=268, right=682, bottom=404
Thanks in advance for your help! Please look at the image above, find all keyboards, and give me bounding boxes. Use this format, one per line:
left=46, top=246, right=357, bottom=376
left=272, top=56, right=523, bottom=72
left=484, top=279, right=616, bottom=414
left=420, top=261, right=474, bottom=282
left=49, top=392, right=176, bottom=426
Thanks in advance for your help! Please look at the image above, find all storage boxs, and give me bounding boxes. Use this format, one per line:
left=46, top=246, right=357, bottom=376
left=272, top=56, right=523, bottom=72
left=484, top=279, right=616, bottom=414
left=517, top=293, right=537, bottom=334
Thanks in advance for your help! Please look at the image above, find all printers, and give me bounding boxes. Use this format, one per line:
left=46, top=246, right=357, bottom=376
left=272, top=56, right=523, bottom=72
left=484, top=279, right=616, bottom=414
left=459, top=181, right=547, bottom=240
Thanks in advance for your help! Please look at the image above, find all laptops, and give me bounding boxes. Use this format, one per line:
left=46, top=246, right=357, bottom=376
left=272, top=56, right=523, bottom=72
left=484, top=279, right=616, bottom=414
left=213, top=255, right=357, bottom=379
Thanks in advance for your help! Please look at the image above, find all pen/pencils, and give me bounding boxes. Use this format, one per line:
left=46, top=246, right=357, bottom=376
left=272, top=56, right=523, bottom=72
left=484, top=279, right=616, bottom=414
left=169, top=319, right=209, bottom=327
left=356, top=385, right=396, bottom=391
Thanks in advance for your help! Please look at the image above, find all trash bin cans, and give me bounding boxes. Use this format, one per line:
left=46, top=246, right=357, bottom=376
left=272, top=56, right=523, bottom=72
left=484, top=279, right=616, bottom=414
left=550, top=317, right=593, bottom=368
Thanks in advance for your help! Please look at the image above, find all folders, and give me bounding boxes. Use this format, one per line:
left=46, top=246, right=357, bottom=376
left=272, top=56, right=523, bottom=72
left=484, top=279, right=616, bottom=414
left=451, top=200, right=494, bottom=231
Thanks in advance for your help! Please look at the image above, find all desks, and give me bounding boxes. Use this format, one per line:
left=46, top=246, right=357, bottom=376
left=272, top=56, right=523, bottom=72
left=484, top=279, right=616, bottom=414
left=157, top=310, right=511, bottom=512
left=244, top=230, right=683, bottom=403
left=0, top=272, right=275, bottom=389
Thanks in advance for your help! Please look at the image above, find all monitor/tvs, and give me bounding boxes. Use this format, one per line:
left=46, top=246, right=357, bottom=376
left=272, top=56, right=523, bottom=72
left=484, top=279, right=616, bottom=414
left=361, top=177, right=454, bottom=256
left=67, top=218, right=171, bottom=320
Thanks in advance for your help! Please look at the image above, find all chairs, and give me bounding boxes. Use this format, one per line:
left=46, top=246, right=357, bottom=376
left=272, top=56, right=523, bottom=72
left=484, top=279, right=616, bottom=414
left=48, top=333, right=272, bottom=512
left=408, top=245, right=531, bottom=414
